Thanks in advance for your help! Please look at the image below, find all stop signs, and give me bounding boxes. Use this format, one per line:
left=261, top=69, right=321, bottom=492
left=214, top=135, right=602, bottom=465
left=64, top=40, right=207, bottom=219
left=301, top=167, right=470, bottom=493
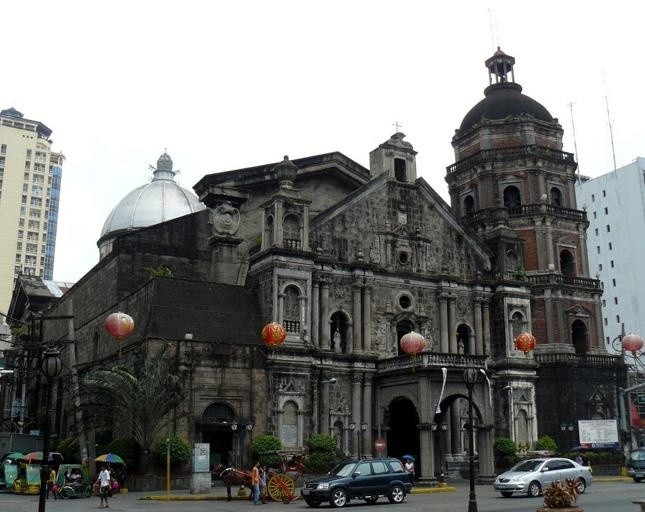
left=375, top=438, right=385, bottom=451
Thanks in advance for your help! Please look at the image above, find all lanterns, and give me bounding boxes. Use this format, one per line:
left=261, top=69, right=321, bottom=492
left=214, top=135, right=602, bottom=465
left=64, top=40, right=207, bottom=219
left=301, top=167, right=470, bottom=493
left=621, top=333, right=644, bottom=359
left=399, top=331, right=427, bottom=374
left=103, top=312, right=135, bottom=360
left=515, top=331, right=537, bottom=359
left=260, top=322, right=287, bottom=360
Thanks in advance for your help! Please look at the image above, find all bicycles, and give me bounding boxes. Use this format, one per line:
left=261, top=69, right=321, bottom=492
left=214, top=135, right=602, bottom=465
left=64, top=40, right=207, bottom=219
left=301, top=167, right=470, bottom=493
left=56, top=481, right=93, bottom=499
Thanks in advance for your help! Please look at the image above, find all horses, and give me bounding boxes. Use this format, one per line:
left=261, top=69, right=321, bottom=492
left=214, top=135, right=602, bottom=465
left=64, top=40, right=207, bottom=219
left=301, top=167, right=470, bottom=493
left=210, top=459, right=255, bottom=503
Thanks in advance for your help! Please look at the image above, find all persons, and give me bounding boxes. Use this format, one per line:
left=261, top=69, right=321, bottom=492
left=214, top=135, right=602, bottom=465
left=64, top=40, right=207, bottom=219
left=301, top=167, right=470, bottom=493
left=576, top=450, right=584, bottom=467
left=332, top=327, right=343, bottom=353
left=93, top=464, right=112, bottom=508
left=45, top=465, right=59, bottom=500
left=252, top=460, right=264, bottom=506
left=405, top=459, right=415, bottom=484
left=259, top=464, right=269, bottom=506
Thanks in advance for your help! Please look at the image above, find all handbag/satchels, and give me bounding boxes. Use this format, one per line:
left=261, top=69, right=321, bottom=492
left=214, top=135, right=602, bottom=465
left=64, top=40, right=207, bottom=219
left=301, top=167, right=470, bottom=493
left=51, top=484, right=58, bottom=493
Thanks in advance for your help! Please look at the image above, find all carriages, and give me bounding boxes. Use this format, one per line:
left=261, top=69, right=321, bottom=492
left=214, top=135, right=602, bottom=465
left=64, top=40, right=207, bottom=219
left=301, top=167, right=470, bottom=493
left=210, top=450, right=305, bottom=502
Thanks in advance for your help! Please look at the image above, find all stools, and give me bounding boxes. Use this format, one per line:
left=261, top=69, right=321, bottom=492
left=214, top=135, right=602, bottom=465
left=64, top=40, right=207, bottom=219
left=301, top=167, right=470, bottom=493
left=632, top=500, right=645, bottom=512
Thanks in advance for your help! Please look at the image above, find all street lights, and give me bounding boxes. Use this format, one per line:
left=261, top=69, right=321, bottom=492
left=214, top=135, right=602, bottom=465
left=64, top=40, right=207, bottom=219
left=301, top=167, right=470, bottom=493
left=431, top=423, right=448, bottom=488
left=560, top=423, right=574, bottom=458
left=230, top=416, right=253, bottom=497
left=38, top=344, right=62, bottom=512
left=349, top=422, right=368, bottom=461
left=463, top=368, right=478, bottom=512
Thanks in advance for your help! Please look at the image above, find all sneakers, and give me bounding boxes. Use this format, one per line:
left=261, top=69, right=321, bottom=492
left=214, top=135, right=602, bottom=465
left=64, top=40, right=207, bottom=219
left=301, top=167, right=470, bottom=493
left=253, top=501, right=269, bottom=506
left=97, top=505, right=109, bottom=509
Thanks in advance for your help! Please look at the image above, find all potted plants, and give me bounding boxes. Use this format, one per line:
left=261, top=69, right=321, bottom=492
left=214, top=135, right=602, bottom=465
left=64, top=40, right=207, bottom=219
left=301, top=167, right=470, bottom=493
left=536, top=476, right=585, bottom=512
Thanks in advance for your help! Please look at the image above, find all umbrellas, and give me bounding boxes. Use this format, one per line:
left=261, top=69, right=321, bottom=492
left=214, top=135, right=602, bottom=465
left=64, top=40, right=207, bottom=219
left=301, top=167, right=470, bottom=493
left=6, top=452, right=24, bottom=460
left=93, top=452, right=125, bottom=469
left=401, top=455, right=416, bottom=462
left=22, top=451, right=44, bottom=461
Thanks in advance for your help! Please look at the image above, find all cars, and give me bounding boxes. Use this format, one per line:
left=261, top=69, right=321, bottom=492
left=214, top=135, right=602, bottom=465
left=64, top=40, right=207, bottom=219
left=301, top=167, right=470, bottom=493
left=627, top=447, right=645, bottom=483
left=572, top=442, right=620, bottom=452
left=300, top=457, right=412, bottom=508
left=493, top=458, right=593, bottom=498
